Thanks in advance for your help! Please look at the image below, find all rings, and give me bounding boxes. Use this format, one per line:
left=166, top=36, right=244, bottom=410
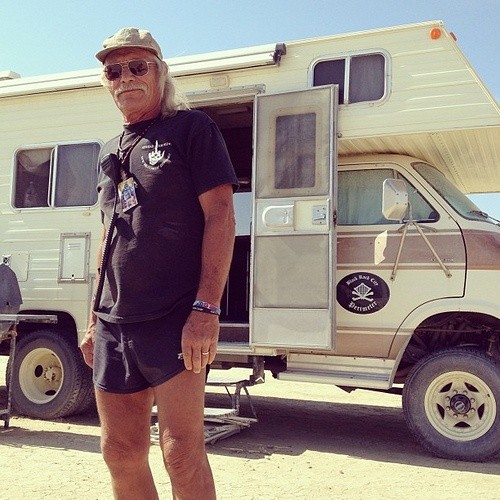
left=202, top=352, right=209, bottom=356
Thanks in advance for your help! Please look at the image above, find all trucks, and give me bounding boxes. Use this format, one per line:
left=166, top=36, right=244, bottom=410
left=0, top=21, right=500, bottom=465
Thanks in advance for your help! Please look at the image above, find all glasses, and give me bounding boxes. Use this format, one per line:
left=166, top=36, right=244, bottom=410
left=102, top=60, right=156, bottom=81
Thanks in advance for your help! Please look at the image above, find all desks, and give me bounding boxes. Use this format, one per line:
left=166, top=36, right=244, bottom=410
left=0, top=313, right=56, bottom=427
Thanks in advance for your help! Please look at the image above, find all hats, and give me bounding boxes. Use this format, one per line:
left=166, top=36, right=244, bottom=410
left=96, top=27, right=163, bottom=60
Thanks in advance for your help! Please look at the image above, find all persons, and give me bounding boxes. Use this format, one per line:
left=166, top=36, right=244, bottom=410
left=80, top=28, right=240, bottom=500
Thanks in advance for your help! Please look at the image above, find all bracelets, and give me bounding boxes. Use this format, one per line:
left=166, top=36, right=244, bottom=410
left=193, top=301, right=221, bottom=315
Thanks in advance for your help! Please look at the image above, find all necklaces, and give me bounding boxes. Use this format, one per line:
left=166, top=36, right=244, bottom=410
left=114, top=119, right=155, bottom=165
